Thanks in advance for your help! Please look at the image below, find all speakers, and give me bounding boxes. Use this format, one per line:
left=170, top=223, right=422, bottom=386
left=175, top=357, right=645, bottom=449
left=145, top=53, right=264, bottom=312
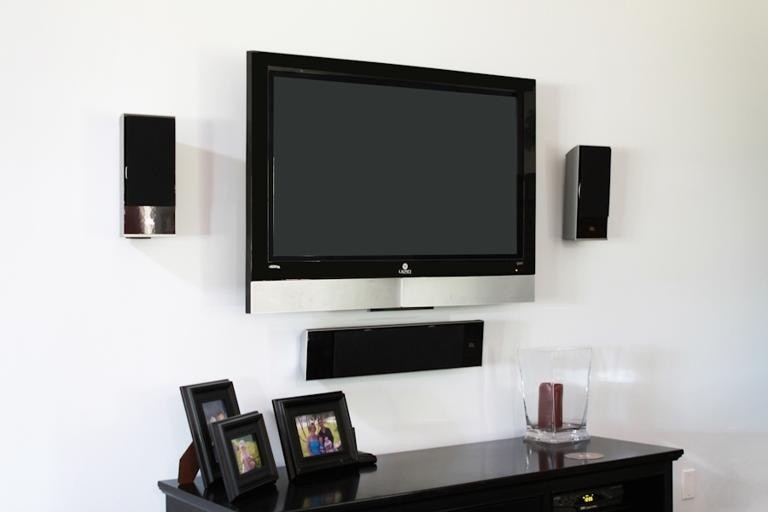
left=119, top=113, right=175, bottom=239
left=303, top=319, right=483, bottom=382
left=563, top=144, right=611, bottom=241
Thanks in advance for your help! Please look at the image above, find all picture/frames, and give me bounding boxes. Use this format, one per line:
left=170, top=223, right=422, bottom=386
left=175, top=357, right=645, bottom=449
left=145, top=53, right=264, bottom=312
left=178, top=378, right=361, bottom=503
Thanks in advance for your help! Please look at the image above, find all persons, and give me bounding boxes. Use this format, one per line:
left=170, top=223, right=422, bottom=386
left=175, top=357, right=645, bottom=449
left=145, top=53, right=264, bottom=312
left=239, top=440, right=256, bottom=473
left=308, top=418, right=334, bottom=456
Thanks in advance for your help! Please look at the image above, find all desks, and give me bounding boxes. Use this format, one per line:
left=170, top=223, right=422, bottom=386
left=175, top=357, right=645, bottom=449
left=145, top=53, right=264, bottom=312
left=155, top=437, right=684, bottom=510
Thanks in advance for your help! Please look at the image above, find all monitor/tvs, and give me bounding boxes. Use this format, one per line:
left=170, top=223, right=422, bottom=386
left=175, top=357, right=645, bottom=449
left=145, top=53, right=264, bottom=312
left=245, top=50, right=536, bottom=314
